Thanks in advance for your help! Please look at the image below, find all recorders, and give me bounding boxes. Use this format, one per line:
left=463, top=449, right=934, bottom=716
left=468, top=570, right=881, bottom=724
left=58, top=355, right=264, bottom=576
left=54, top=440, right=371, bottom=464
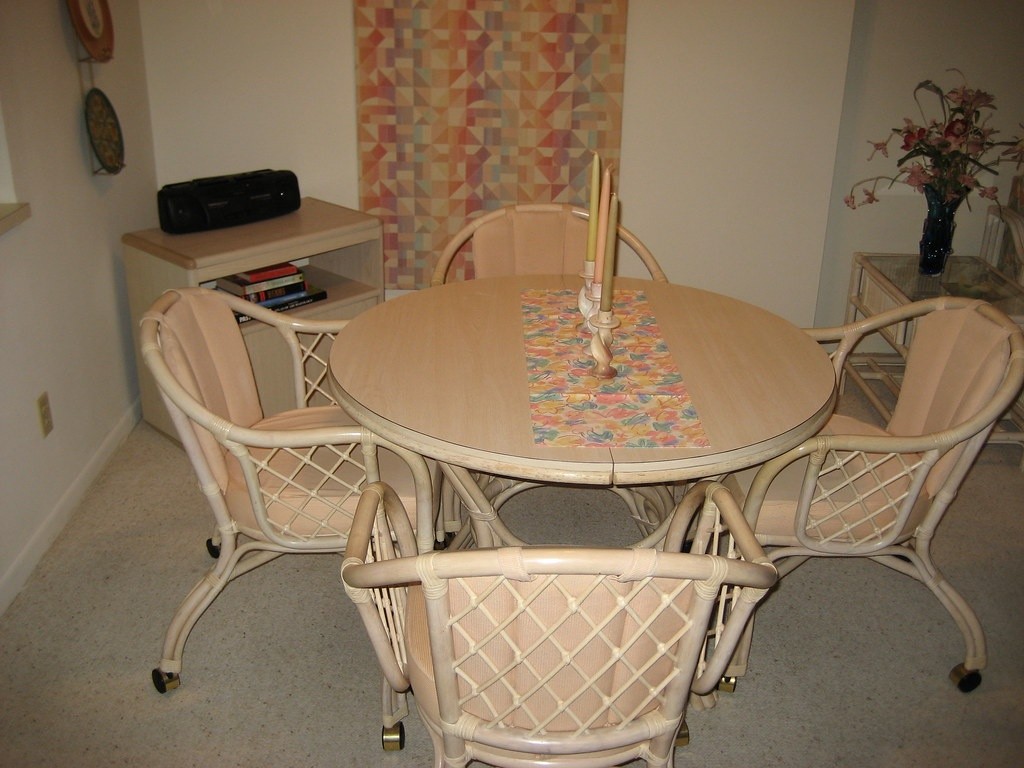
left=156, top=169, right=301, bottom=236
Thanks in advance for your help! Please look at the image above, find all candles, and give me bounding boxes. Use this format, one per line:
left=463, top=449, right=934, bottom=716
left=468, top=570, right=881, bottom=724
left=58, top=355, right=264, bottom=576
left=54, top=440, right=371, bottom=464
left=594, top=163, right=614, bottom=283
left=585, top=151, right=599, bottom=261
left=600, top=193, right=618, bottom=311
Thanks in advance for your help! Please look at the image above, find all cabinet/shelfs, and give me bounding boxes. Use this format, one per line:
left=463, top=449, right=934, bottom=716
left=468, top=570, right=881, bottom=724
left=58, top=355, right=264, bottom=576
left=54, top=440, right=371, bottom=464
left=121, top=196, right=385, bottom=456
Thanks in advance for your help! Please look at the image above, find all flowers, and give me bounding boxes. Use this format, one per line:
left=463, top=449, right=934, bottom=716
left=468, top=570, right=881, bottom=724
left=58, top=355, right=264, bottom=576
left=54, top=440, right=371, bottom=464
left=843, top=69, right=1024, bottom=274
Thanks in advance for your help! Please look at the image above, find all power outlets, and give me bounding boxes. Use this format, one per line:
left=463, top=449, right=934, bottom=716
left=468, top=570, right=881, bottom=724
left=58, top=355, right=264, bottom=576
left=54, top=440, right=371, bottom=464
left=36, top=392, right=53, bottom=438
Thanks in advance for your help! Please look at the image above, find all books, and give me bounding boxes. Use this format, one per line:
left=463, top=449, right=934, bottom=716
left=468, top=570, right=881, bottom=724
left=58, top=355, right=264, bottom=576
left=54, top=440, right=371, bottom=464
left=215, top=261, right=328, bottom=325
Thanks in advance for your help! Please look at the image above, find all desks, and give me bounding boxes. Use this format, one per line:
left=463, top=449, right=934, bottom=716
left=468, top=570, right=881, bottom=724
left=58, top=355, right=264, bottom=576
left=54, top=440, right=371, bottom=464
left=329, top=274, right=838, bottom=713
left=838, top=252, right=1024, bottom=466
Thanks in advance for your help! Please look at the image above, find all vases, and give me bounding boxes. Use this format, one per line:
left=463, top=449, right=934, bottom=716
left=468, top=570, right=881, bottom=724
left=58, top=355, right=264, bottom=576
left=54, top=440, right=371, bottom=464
left=919, top=184, right=973, bottom=274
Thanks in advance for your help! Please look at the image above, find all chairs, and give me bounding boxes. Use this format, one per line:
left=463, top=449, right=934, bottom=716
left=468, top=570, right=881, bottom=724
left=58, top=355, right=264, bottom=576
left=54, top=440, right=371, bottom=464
left=136, top=202, right=1023, bottom=768
